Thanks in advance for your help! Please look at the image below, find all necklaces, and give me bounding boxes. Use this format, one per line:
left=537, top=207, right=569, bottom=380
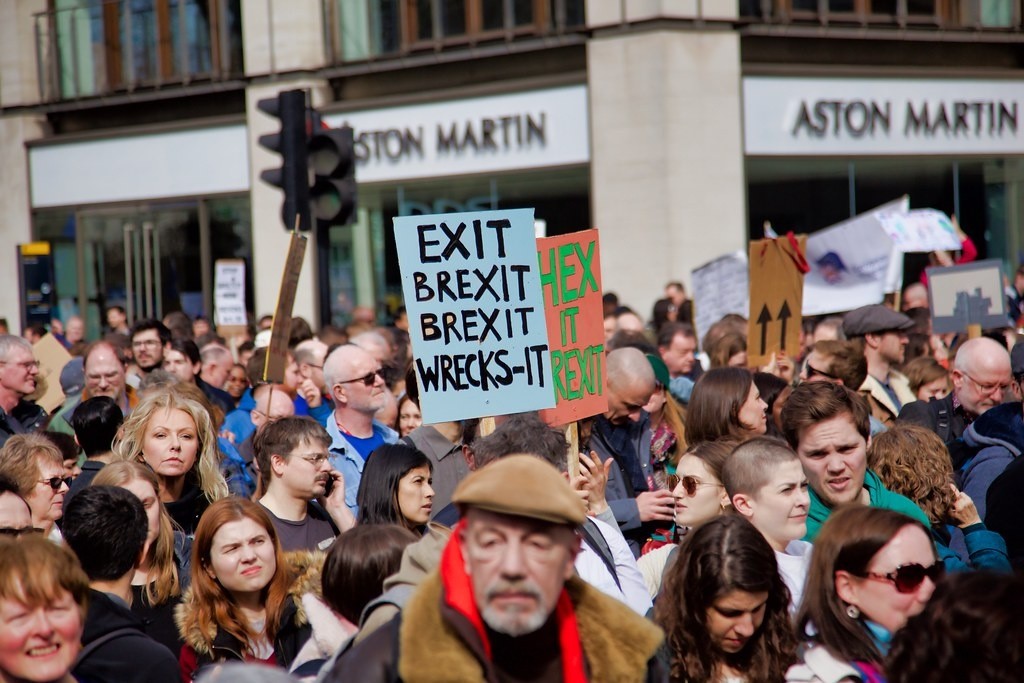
left=337, top=422, right=352, bottom=436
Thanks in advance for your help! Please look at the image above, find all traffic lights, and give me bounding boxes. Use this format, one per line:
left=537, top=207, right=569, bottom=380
left=307, top=125, right=360, bottom=227
left=255, top=88, right=311, bottom=233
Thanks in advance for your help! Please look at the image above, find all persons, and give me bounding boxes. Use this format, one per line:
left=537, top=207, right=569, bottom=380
left=321, top=452, right=669, bottom=683
left=0, top=218, right=1024, bottom=683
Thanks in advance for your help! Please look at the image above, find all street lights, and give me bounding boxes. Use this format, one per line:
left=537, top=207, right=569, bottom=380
left=15, top=242, right=51, bottom=336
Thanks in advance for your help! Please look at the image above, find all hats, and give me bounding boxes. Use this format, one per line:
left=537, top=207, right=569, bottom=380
left=449, top=454, right=587, bottom=527
left=843, top=304, right=915, bottom=340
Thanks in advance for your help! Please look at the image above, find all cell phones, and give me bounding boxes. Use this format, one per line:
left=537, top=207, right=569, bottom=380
left=323, top=475, right=335, bottom=497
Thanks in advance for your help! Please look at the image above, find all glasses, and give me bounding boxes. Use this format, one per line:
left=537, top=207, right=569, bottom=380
left=840, top=562, right=946, bottom=594
left=132, top=339, right=162, bottom=349
left=37, top=477, right=73, bottom=490
left=339, top=368, right=385, bottom=387
left=806, top=360, right=835, bottom=382
left=1, top=359, right=39, bottom=369
left=666, top=473, right=725, bottom=496
left=964, top=371, right=1013, bottom=395
left=275, top=453, right=331, bottom=464
